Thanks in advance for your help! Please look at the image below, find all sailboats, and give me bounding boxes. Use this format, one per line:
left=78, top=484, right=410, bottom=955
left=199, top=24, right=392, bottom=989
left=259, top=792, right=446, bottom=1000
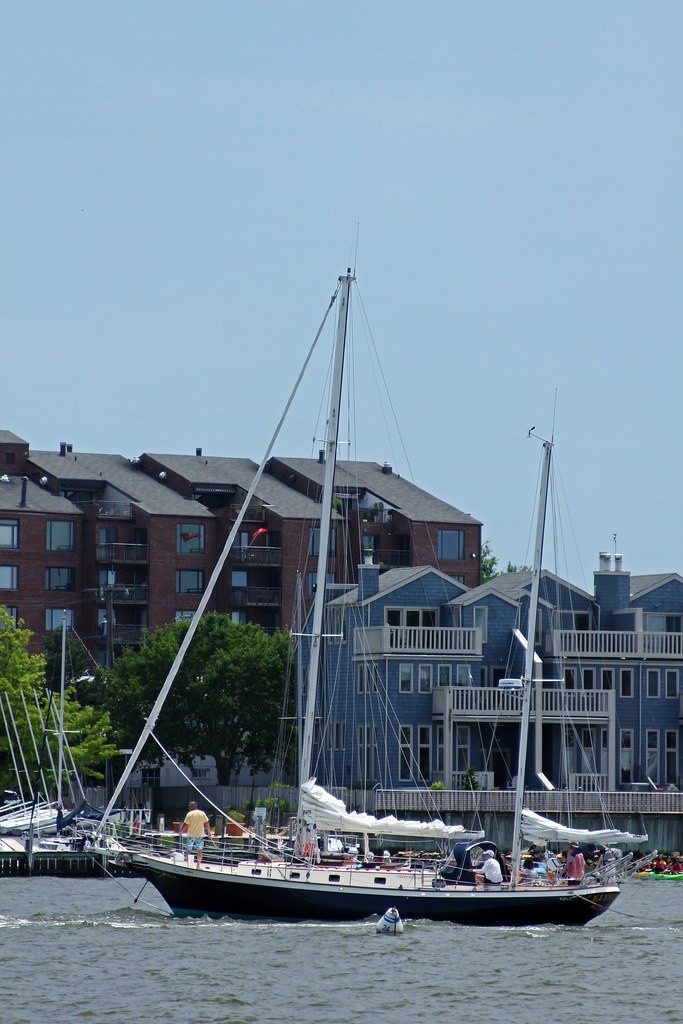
left=0, top=606, right=126, bottom=837
left=84, top=221, right=659, bottom=929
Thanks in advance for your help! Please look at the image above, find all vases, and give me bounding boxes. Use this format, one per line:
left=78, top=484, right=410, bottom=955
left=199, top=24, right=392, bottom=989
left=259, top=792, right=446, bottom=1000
left=173, top=822, right=188, bottom=833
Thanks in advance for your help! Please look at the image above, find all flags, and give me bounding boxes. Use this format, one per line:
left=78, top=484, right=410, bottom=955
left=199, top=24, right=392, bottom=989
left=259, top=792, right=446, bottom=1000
left=183, top=532, right=200, bottom=541
left=252, top=528, right=269, bottom=539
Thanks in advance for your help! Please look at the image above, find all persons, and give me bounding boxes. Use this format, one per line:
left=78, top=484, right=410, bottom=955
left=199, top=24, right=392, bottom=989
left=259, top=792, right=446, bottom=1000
left=518, top=859, right=539, bottom=884
left=469, top=849, right=503, bottom=887
left=178, top=801, right=212, bottom=868
left=559, top=842, right=605, bottom=885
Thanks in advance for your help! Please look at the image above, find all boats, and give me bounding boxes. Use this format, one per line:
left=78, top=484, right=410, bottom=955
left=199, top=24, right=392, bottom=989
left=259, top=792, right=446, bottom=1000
left=632, top=870, right=683, bottom=883
left=299, top=783, right=485, bottom=856
left=518, top=805, right=653, bottom=863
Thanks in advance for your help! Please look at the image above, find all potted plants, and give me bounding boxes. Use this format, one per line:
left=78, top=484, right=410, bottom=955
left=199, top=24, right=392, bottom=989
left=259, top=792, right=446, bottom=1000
left=226, top=811, right=246, bottom=836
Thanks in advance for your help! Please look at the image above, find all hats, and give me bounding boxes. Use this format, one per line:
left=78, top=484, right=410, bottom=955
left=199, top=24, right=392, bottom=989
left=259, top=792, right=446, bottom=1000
left=483, top=850, right=494, bottom=858
left=571, top=841, right=579, bottom=848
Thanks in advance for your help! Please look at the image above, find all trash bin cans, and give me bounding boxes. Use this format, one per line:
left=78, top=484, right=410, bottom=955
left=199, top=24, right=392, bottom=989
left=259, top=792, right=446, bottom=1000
left=215, top=815, right=223, bottom=835
left=156, top=814, right=165, bottom=831
